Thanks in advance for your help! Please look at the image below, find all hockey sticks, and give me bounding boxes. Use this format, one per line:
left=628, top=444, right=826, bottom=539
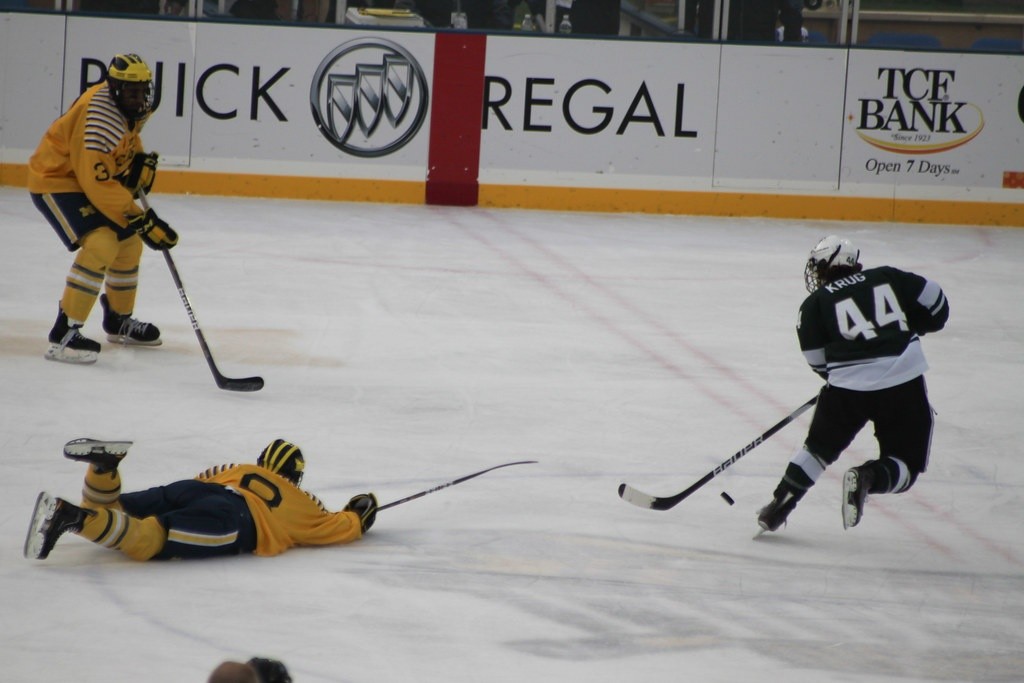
left=137, top=186, right=264, bottom=392
left=618, top=395, right=819, bottom=511
left=377, top=460, right=536, bottom=512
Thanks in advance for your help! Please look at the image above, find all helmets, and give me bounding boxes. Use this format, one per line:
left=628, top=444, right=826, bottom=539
left=257, top=439, right=305, bottom=490
left=106, top=53, right=152, bottom=118
left=805, top=236, right=859, bottom=293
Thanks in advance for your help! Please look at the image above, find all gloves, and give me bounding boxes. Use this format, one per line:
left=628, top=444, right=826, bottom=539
left=344, top=493, right=378, bottom=532
left=123, top=152, right=158, bottom=199
left=128, top=208, right=178, bottom=251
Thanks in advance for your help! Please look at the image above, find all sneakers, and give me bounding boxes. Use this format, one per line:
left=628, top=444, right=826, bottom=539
left=841, top=461, right=875, bottom=531
left=64, top=439, right=133, bottom=480
left=24, top=492, right=98, bottom=560
left=44, top=300, right=100, bottom=363
left=753, top=490, right=796, bottom=540
left=100, top=294, right=161, bottom=345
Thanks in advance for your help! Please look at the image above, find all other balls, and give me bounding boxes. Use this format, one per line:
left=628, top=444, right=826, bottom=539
left=720, top=492, right=735, bottom=506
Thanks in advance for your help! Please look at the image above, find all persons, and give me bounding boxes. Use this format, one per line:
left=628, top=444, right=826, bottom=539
left=752, top=235, right=950, bottom=539
left=80, top=0, right=282, bottom=21
left=28, top=53, right=179, bottom=364
left=348, top=0, right=546, bottom=31
left=569, top=0, right=621, bottom=36
left=23, top=438, right=378, bottom=561
left=685, top=0, right=811, bottom=43
left=209, top=657, right=291, bottom=683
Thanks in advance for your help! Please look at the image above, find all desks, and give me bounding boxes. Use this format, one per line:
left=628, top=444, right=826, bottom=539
left=345, top=7, right=422, bottom=27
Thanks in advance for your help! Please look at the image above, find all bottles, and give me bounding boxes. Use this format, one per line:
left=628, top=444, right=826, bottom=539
left=559, top=14, right=572, bottom=34
left=522, top=15, right=533, bottom=31
